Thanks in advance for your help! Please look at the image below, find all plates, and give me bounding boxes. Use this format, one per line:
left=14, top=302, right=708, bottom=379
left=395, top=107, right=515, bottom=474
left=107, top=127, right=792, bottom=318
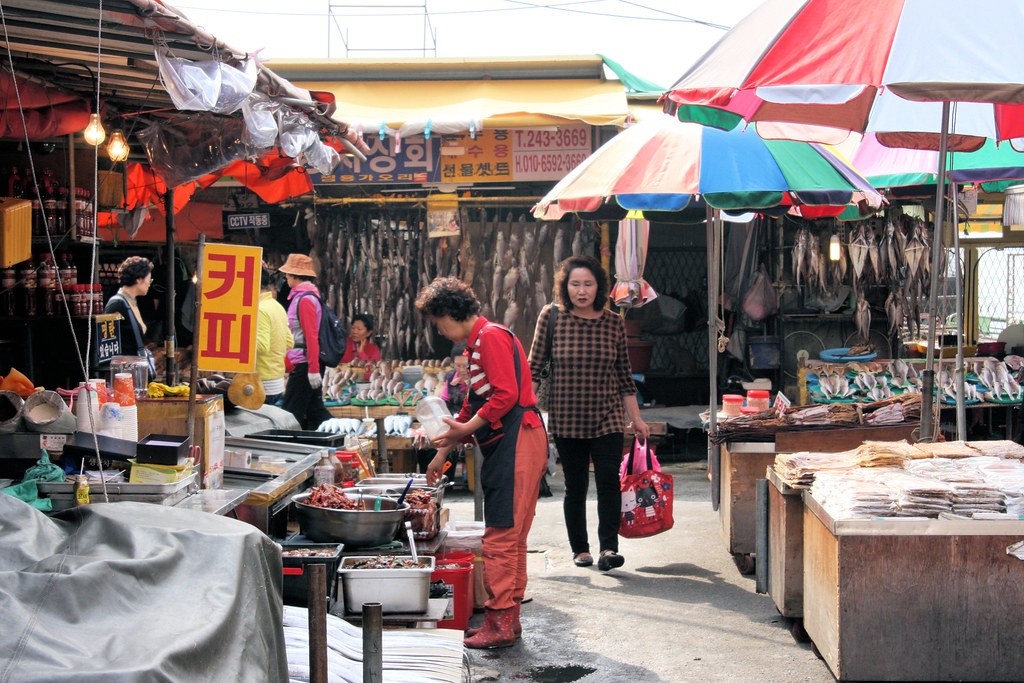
left=820, top=348, right=877, bottom=362
left=807, top=368, right=917, bottom=404
left=919, top=368, right=1024, bottom=404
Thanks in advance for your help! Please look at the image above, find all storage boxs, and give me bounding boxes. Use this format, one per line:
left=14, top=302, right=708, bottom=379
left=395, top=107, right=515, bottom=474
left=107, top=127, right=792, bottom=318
left=137, top=433, right=189, bottom=466
left=244, top=429, right=346, bottom=447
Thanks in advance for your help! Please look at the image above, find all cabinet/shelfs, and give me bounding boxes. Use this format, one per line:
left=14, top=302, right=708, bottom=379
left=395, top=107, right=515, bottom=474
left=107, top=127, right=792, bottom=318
left=0, top=135, right=105, bottom=388
left=326, top=359, right=474, bottom=493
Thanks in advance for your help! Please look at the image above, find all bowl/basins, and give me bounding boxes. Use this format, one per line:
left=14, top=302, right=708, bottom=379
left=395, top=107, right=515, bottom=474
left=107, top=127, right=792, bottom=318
left=341, top=486, right=437, bottom=503
left=290, top=493, right=411, bottom=547
left=625, top=320, right=644, bottom=337
left=626, top=341, right=656, bottom=372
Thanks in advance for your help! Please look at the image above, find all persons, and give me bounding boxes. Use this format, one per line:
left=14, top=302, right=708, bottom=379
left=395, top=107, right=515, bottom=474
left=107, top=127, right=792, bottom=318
left=528, top=257, right=649, bottom=572
left=438, top=348, right=468, bottom=415
left=341, top=317, right=380, bottom=363
left=256, top=269, right=294, bottom=405
left=417, top=278, right=549, bottom=648
left=105, top=257, right=155, bottom=357
left=279, top=254, right=333, bottom=430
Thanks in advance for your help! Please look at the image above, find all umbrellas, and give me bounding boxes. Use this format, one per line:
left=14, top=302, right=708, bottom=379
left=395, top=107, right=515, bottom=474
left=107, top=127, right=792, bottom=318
left=599, top=0, right=1024, bottom=441
left=611, top=218, right=658, bottom=319
left=532, top=118, right=887, bottom=429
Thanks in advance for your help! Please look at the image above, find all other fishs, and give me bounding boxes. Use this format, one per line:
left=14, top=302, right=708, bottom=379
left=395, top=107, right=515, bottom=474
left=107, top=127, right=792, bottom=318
left=317, top=415, right=412, bottom=435
left=934, top=355, right=1024, bottom=403
left=815, top=358, right=922, bottom=401
left=793, top=219, right=948, bottom=339
left=302, top=207, right=585, bottom=353
left=327, top=356, right=449, bottom=401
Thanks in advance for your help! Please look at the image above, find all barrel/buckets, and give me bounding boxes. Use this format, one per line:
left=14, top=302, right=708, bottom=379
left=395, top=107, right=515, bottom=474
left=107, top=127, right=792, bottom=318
left=748, top=337, right=781, bottom=369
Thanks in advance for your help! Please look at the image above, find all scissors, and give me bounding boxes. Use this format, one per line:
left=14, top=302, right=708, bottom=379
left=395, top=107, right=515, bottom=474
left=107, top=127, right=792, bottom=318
left=189, top=445, right=201, bottom=465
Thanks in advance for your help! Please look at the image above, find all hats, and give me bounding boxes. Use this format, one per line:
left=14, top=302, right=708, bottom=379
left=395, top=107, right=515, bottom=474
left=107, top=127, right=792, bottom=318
left=276, top=254, right=317, bottom=277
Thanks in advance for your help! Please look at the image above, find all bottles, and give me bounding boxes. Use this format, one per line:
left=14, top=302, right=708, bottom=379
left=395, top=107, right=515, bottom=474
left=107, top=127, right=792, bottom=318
left=402, top=495, right=441, bottom=539
left=742, top=409, right=759, bottom=418
left=3, top=167, right=69, bottom=236
left=336, top=450, right=359, bottom=489
left=747, top=390, right=770, bottom=412
left=76, top=186, right=94, bottom=236
left=110, top=355, right=149, bottom=397
left=42, top=253, right=77, bottom=315
left=1, top=254, right=37, bottom=315
left=723, top=395, right=744, bottom=417
left=73, top=284, right=87, bottom=316
left=313, top=452, right=334, bottom=486
left=87, top=284, right=103, bottom=314
left=73, top=475, right=89, bottom=507
left=328, top=449, right=345, bottom=487
left=416, top=396, right=454, bottom=443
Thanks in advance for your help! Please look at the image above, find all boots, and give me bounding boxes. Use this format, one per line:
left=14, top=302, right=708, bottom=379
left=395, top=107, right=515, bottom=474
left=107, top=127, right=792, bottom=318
left=462, top=606, right=515, bottom=649
left=466, top=603, right=523, bottom=640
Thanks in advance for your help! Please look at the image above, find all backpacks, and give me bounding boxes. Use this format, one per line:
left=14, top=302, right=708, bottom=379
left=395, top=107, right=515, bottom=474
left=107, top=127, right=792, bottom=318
left=296, top=290, right=347, bottom=368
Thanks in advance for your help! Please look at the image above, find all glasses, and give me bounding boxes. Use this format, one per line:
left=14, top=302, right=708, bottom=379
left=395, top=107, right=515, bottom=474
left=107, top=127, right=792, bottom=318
left=141, top=278, right=154, bottom=283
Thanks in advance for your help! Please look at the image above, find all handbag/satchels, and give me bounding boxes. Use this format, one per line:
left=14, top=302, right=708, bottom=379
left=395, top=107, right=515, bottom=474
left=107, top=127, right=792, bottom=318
left=138, top=347, right=157, bottom=383
left=619, top=432, right=676, bottom=536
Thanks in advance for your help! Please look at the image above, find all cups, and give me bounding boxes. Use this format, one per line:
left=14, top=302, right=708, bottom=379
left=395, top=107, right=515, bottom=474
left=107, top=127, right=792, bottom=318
left=76, top=373, right=139, bottom=443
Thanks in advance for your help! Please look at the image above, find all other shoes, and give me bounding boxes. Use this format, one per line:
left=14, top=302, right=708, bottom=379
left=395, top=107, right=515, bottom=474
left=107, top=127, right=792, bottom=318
left=573, top=551, right=593, bottom=567
left=597, top=549, right=625, bottom=571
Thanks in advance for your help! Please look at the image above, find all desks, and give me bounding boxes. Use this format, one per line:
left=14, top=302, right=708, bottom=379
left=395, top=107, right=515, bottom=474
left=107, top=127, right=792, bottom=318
left=693, top=403, right=1024, bottom=683
